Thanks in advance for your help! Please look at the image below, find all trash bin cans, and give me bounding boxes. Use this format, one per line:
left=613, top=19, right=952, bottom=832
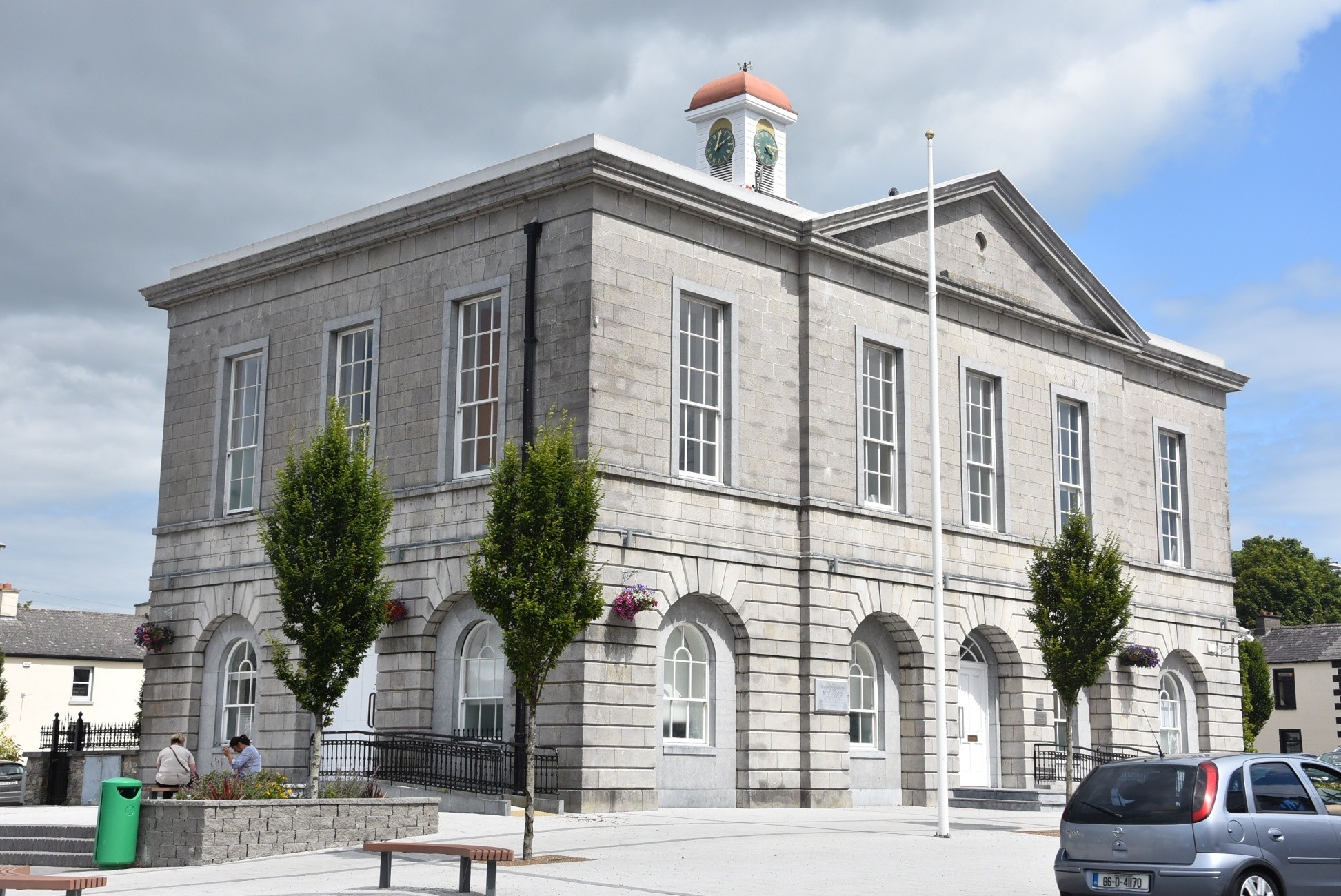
left=93, top=777, right=143, bottom=869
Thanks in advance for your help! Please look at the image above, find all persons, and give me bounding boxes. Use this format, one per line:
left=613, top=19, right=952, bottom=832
left=150, top=734, right=197, bottom=799
left=1278, top=776, right=1341, bottom=816
left=222, top=734, right=262, bottom=779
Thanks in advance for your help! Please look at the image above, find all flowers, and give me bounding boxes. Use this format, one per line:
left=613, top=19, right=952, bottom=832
left=385, top=599, right=406, bottom=624
left=614, top=584, right=658, bottom=621
left=1120, top=646, right=1160, bottom=668
left=134, top=625, right=174, bottom=654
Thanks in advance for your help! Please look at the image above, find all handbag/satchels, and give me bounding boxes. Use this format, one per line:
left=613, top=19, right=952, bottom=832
left=187, top=771, right=200, bottom=788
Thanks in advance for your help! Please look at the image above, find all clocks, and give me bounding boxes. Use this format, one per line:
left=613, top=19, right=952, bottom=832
left=753, top=129, right=778, bottom=167
left=705, top=128, right=735, bottom=166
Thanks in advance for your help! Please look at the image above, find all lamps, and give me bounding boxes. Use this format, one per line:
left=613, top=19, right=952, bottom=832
left=624, top=531, right=635, bottom=548
left=164, top=575, right=174, bottom=590
left=831, top=556, right=840, bottom=574
left=943, top=574, right=949, bottom=590
left=395, top=547, right=404, bottom=564
left=1221, top=617, right=1230, bottom=630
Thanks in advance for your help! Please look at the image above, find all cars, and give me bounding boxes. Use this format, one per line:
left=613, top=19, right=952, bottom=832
left=1051, top=748, right=1340, bottom=896
left=0, top=760, right=25, bottom=805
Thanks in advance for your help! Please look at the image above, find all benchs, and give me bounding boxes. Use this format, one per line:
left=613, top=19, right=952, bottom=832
left=363, top=842, right=515, bottom=896
left=142, top=787, right=180, bottom=800
left=0, top=866, right=117, bottom=896
